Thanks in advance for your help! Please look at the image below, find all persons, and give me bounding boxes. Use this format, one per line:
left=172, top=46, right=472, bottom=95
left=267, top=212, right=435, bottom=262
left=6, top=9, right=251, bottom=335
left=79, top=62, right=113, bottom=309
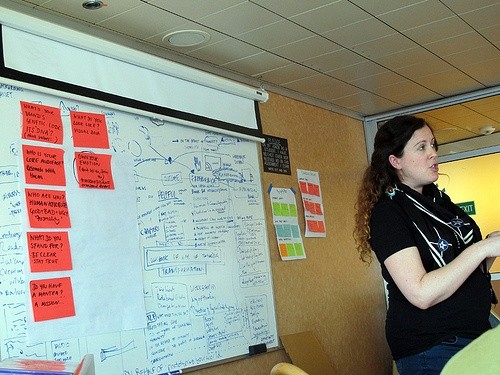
left=353, top=115, right=500, bottom=375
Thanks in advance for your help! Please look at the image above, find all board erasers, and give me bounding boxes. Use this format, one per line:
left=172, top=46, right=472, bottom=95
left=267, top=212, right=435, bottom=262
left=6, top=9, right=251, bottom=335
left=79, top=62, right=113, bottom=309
left=248, top=343, right=267, bottom=357
